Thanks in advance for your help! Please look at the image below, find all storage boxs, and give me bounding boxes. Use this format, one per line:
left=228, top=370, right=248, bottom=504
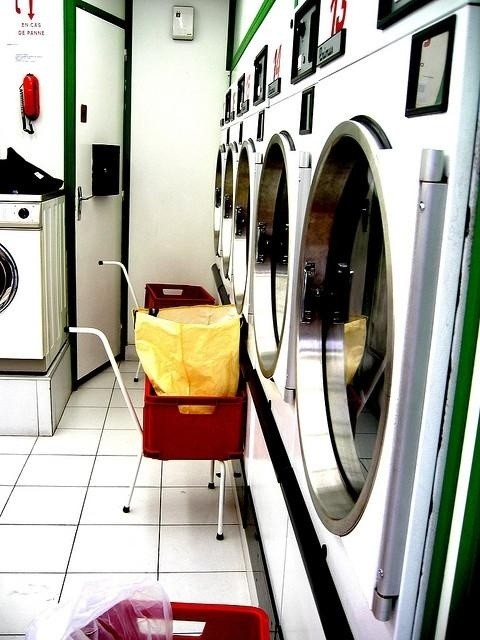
left=142, top=364, right=247, bottom=459
left=68, top=599, right=271, bottom=640
left=144, top=284, right=215, bottom=309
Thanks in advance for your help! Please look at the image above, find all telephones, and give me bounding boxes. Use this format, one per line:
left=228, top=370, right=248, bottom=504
left=18, top=74, right=40, bottom=125
